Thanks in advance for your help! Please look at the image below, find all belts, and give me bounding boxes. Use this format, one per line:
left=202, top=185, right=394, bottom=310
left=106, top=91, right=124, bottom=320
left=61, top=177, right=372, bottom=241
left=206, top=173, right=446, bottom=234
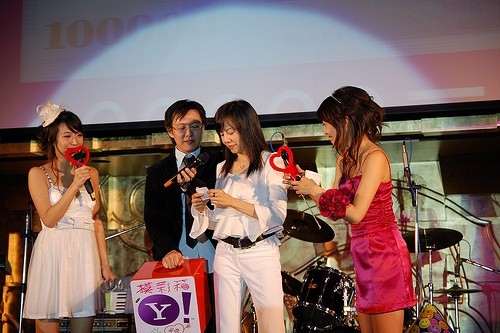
left=221, top=232, right=275, bottom=251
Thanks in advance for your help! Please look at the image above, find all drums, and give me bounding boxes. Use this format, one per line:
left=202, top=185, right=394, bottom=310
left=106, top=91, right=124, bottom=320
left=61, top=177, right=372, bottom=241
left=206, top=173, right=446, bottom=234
left=291, top=265, right=356, bottom=327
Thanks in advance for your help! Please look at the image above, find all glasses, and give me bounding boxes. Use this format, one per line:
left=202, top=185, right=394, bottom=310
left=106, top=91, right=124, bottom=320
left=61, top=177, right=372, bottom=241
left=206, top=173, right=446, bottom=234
left=171, top=123, right=205, bottom=134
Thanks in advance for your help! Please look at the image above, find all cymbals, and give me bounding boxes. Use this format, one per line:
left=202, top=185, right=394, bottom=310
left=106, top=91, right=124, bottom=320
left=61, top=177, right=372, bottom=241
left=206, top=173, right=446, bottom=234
left=281, top=272, right=303, bottom=299
left=428, top=286, right=484, bottom=298
left=402, top=227, right=462, bottom=254
left=282, top=209, right=335, bottom=243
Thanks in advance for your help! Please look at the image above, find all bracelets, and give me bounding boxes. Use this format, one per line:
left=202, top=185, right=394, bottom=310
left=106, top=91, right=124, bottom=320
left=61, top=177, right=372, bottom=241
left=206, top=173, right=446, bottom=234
left=101, top=264, right=111, bottom=269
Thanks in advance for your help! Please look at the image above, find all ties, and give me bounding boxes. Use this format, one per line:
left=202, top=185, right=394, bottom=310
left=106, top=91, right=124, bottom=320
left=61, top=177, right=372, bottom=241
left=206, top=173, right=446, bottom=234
left=183, top=155, right=199, bottom=248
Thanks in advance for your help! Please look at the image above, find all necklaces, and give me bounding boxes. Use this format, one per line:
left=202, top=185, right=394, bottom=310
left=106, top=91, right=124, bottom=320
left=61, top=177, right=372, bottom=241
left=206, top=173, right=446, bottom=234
left=239, top=159, right=249, bottom=168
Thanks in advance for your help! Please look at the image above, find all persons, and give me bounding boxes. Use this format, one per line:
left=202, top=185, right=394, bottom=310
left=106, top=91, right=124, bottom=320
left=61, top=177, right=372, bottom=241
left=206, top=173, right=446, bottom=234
left=23, top=99, right=120, bottom=333
left=143, top=99, right=226, bottom=333
left=188, top=100, right=287, bottom=333
left=282, top=86, right=418, bottom=333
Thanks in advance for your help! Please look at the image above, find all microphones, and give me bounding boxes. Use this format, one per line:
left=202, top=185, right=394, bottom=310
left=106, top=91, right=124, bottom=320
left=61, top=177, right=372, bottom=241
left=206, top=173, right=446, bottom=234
left=282, top=134, right=288, bottom=146
left=164, top=152, right=210, bottom=187
left=454, top=243, right=461, bottom=277
left=73, top=153, right=96, bottom=200
left=402, top=145, right=408, bottom=170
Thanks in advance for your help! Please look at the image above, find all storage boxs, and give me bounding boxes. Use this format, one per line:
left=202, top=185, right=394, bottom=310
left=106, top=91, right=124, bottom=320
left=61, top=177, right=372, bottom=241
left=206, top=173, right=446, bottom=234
left=130, top=258, right=212, bottom=333
